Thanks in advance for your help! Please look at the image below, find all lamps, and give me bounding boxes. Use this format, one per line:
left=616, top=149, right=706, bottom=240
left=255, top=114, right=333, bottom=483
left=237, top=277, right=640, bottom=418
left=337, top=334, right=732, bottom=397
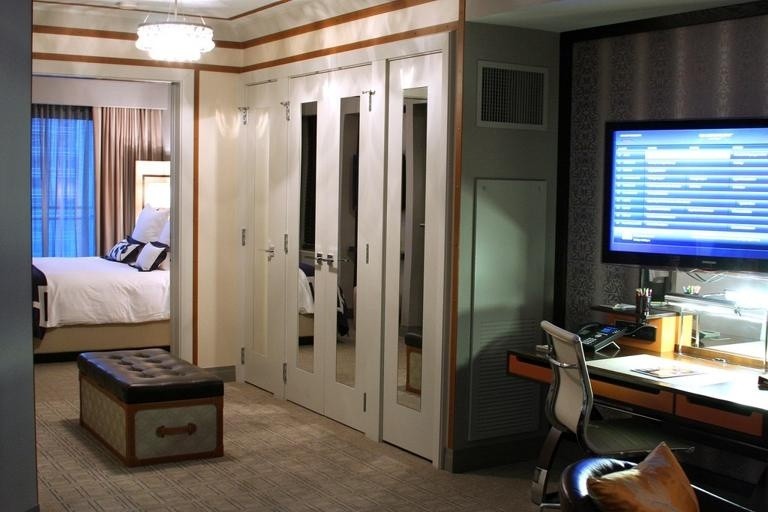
left=134, top=0, right=215, bottom=62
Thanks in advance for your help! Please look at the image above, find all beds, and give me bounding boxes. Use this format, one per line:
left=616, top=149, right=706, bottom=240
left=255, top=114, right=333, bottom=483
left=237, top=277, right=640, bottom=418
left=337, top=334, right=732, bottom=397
left=32, top=256, right=170, bottom=363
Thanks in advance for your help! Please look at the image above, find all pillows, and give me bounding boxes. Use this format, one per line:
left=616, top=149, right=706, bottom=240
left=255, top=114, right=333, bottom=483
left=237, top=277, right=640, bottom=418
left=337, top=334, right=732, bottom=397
left=100, top=202, right=170, bottom=272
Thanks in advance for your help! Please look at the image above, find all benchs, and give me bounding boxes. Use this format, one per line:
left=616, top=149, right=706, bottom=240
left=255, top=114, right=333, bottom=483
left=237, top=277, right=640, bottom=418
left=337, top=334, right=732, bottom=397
left=76, top=347, right=223, bottom=468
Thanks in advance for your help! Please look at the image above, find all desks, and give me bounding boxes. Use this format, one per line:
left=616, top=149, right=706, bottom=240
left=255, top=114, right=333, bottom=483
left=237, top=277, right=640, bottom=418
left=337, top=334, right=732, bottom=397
left=505, top=342, right=767, bottom=512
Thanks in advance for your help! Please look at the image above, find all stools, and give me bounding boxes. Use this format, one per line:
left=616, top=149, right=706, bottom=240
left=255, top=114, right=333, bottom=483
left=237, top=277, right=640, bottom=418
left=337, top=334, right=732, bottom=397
left=404, top=330, right=421, bottom=394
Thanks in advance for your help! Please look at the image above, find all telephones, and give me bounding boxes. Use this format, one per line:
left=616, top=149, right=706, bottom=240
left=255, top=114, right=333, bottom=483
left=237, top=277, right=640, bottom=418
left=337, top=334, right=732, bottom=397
left=576, top=323, right=627, bottom=351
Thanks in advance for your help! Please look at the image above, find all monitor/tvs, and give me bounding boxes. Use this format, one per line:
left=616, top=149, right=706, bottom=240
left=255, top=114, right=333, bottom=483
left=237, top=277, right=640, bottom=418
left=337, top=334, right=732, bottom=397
left=599, top=115, right=768, bottom=273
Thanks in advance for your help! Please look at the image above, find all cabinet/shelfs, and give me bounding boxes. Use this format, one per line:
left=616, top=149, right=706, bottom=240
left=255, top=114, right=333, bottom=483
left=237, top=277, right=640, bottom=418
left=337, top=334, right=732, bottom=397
left=235, top=30, right=387, bottom=445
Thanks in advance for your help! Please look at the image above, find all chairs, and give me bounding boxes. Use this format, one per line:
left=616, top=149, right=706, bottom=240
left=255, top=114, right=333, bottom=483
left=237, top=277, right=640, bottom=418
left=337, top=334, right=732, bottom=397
left=530, top=320, right=755, bottom=512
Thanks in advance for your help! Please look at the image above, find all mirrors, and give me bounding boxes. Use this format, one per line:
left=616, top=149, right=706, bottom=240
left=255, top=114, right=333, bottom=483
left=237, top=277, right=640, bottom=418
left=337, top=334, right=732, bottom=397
left=380, top=51, right=441, bottom=470
left=637, top=265, right=767, bottom=370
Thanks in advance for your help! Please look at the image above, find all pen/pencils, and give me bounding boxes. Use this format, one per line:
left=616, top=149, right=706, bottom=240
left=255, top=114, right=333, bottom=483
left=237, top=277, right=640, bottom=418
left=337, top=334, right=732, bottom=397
left=635, top=287, right=653, bottom=296
left=682, top=286, right=701, bottom=295
